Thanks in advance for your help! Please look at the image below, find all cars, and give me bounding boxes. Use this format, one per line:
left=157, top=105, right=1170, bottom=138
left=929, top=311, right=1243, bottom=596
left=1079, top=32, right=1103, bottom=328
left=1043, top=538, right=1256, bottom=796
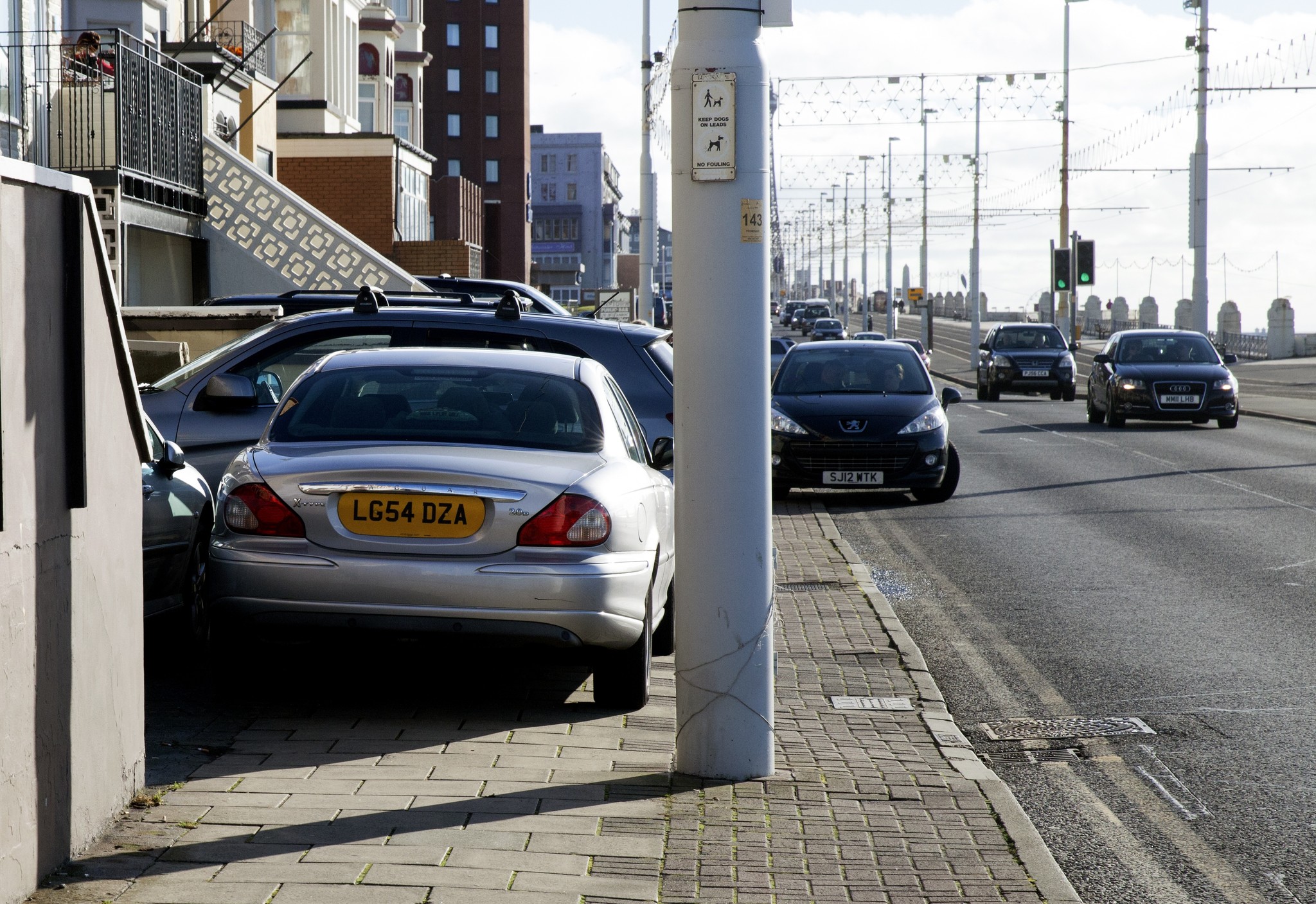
left=883, top=338, right=933, bottom=372
left=770, top=336, right=798, bottom=375
left=141, top=407, right=215, bottom=619
left=771, top=298, right=849, bottom=340
left=1087, top=328, right=1240, bottom=430
left=771, top=338, right=964, bottom=504
left=850, top=332, right=886, bottom=341
left=209, top=346, right=676, bottom=713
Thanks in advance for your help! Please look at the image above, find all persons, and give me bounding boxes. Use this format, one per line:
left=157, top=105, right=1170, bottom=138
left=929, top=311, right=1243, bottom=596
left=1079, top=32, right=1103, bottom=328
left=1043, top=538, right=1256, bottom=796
left=871, top=363, right=905, bottom=390
left=1000, top=333, right=1050, bottom=348
left=800, top=359, right=845, bottom=391
left=67, top=32, right=116, bottom=79
left=1124, top=338, right=1198, bottom=363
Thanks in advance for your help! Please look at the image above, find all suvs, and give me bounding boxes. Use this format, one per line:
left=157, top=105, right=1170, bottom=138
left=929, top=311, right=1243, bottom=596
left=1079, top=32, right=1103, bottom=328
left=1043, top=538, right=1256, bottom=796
left=137, top=268, right=673, bottom=480
left=975, top=321, right=1080, bottom=402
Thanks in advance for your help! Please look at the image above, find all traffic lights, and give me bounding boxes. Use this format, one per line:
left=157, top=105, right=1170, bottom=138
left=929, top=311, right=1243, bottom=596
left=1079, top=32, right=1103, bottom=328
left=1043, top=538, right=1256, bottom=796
left=1052, top=247, right=1072, bottom=292
left=1075, top=239, right=1096, bottom=287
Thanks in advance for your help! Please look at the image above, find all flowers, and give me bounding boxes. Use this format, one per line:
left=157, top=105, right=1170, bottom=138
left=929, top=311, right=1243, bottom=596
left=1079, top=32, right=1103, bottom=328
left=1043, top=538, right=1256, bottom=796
left=224, top=45, right=243, bottom=58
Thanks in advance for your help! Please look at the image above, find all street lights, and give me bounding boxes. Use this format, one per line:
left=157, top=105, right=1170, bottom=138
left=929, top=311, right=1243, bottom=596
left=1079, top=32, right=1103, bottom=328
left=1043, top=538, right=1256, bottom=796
left=808, top=204, right=815, bottom=301
left=794, top=217, right=800, bottom=299
left=885, top=136, right=902, bottom=339
left=862, top=156, right=875, bottom=332
left=801, top=209, right=808, bottom=300
left=919, top=107, right=940, bottom=350
left=819, top=192, right=829, bottom=299
left=1059, top=0, right=1087, bottom=344
left=1184, top=0, right=1209, bottom=338
left=969, top=77, right=994, bottom=369
left=842, top=172, right=855, bottom=326
left=608, top=220, right=615, bottom=290
left=830, top=184, right=840, bottom=316
left=661, top=245, right=666, bottom=298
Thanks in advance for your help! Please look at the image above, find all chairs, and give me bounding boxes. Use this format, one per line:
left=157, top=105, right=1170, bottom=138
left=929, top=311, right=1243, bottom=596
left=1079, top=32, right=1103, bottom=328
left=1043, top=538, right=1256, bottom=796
left=329, top=380, right=579, bottom=435
left=796, top=361, right=825, bottom=385
